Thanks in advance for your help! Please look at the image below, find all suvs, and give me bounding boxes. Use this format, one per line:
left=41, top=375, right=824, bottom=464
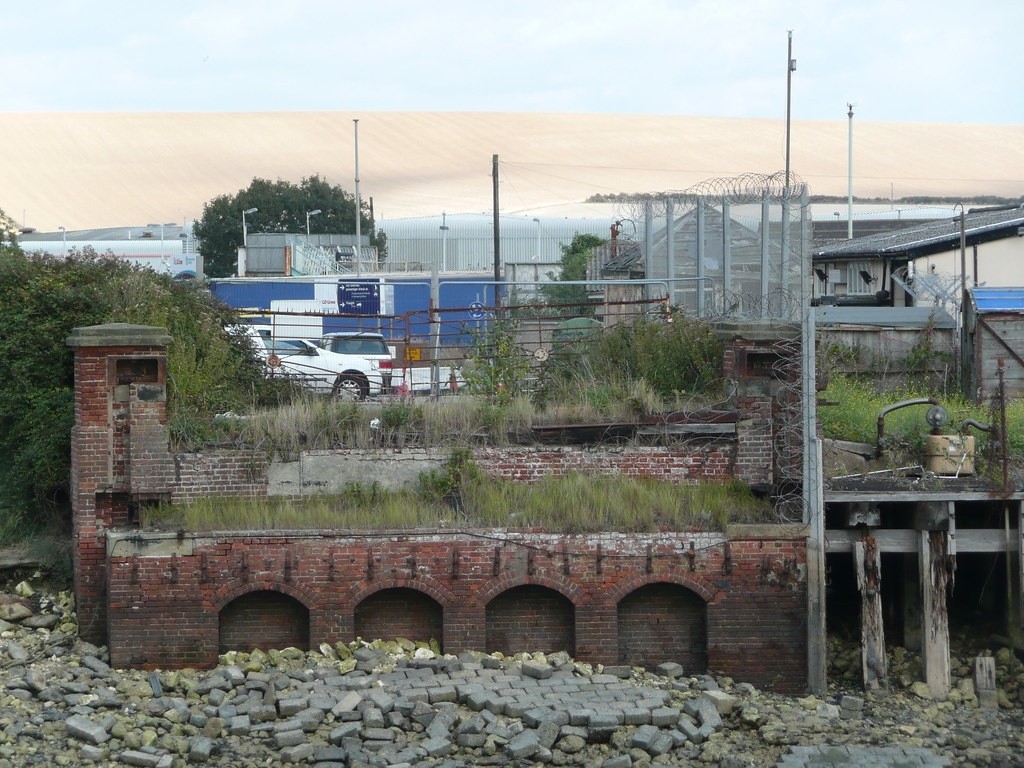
left=319, top=332, right=394, bottom=395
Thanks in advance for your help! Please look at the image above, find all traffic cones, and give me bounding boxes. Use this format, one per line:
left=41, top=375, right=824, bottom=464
left=448, top=373, right=459, bottom=391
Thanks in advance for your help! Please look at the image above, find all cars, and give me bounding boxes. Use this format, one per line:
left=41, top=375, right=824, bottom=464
left=263, top=337, right=384, bottom=403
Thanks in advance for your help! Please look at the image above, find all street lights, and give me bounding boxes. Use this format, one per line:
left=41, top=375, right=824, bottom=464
left=57, top=226, right=68, bottom=249
left=148, top=222, right=175, bottom=260
left=305, top=209, right=323, bottom=242
left=532, top=217, right=544, bottom=264
left=242, top=206, right=259, bottom=246
left=833, top=210, right=842, bottom=229
left=439, top=224, right=449, bottom=272
left=179, top=233, right=189, bottom=264
